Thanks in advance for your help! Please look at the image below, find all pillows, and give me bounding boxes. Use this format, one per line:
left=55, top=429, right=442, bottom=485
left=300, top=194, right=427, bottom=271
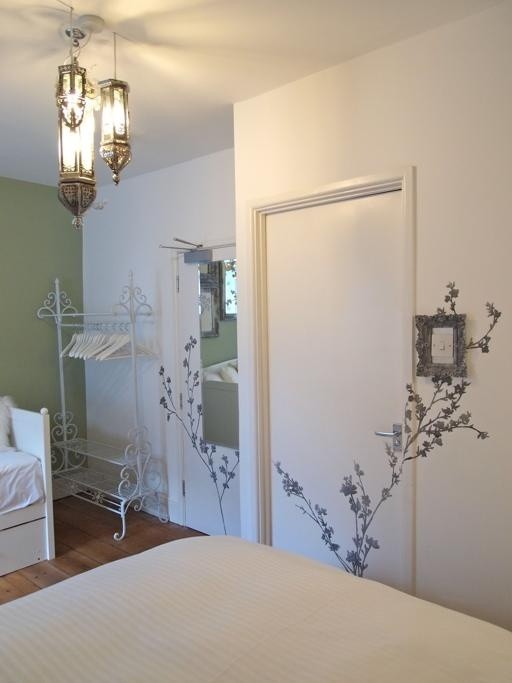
left=203, top=369, right=219, bottom=382
left=219, top=365, right=238, bottom=383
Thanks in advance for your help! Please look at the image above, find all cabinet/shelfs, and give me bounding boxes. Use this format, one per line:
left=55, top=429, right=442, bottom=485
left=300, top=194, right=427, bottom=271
left=34, top=269, right=169, bottom=541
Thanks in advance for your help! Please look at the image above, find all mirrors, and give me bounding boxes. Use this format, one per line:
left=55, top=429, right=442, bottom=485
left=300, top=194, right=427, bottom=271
left=221, top=259, right=238, bottom=320
left=199, top=257, right=238, bottom=451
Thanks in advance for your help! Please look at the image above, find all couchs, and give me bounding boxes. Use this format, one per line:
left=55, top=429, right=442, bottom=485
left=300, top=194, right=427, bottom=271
left=1, top=395, right=55, bottom=575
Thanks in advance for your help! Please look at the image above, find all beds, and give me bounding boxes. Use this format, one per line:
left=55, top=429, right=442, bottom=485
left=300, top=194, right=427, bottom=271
left=201, top=358, right=238, bottom=450
left=1, top=535, right=512, bottom=683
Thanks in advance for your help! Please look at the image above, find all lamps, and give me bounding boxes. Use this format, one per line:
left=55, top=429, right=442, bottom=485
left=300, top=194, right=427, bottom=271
left=52, top=0, right=133, bottom=230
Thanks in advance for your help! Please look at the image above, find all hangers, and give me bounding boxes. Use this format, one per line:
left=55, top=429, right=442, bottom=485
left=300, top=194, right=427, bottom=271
left=60, top=322, right=157, bottom=362
